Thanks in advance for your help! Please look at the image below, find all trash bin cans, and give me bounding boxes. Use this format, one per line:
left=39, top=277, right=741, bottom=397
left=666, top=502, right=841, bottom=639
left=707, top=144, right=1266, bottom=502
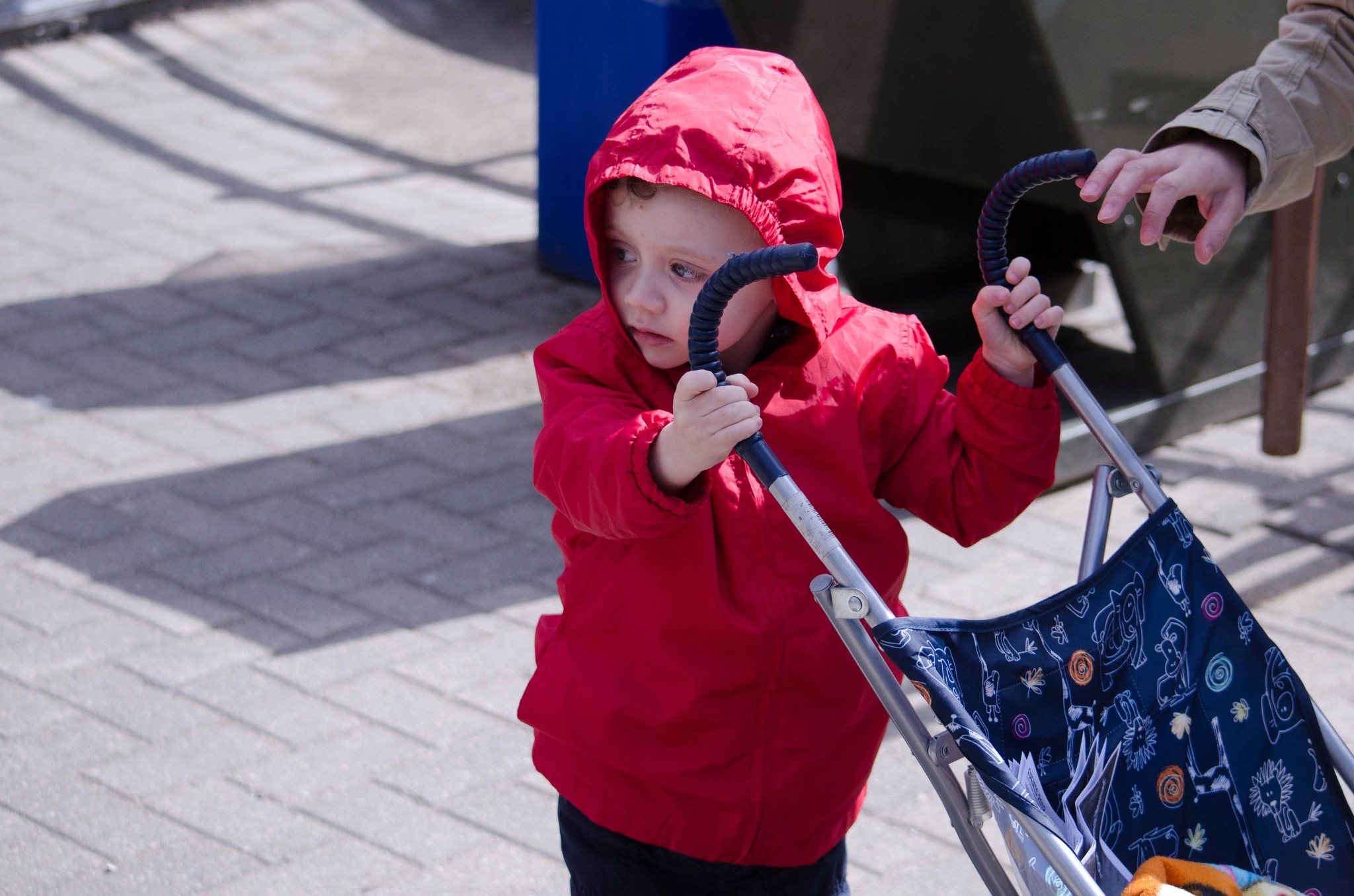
left=539, top=0, right=738, bottom=286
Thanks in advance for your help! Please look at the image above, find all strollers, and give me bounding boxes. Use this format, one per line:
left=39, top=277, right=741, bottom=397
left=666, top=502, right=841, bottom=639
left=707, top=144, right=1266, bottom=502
left=690, top=149, right=1354, bottom=896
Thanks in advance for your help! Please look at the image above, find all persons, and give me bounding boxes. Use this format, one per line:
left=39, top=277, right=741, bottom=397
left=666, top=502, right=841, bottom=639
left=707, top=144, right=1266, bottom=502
left=1072, top=0, right=1354, bottom=265
left=514, top=44, right=1066, bottom=896
